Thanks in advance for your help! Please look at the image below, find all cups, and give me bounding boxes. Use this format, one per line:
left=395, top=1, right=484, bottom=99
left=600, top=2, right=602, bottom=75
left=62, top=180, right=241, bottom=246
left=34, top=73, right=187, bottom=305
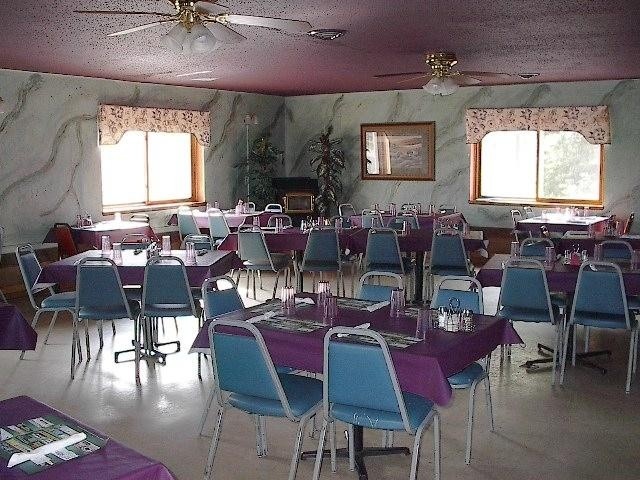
left=72, top=201, right=626, bottom=269
left=272, top=280, right=477, bottom=341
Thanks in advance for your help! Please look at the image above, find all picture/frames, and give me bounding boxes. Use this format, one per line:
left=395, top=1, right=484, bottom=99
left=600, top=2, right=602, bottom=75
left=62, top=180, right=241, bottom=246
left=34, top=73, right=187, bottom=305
left=360, top=119, right=437, bottom=184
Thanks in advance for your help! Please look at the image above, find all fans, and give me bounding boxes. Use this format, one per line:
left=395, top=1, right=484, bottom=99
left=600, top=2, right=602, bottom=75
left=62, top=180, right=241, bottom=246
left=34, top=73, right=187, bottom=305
left=73, top=2, right=313, bottom=38
left=372, top=51, right=542, bottom=91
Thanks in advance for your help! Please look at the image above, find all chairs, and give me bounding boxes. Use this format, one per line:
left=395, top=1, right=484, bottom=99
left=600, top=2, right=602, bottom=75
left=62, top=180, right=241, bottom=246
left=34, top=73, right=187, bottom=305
left=428, top=275, right=493, bottom=466
left=136, top=255, right=206, bottom=381
left=42, top=201, right=483, bottom=301
left=559, top=260, right=637, bottom=395
left=487, top=257, right=566, bottom=385
left=313, top=326, right=441, bottom=479
left=203, top=319, right=336, bottom=478
left=487, top=205, right=639, bottom=373
left=70, top=257, right=140, bottom=379
left=16, top=244, right=83, bottom=362
left=198, top=275, right=245, bottom=436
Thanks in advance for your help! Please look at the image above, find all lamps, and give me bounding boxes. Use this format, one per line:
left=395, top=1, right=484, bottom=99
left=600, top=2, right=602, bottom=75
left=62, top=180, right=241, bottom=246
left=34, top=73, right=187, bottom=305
left=421, top=76, right=458, bottom=96
left=161, top=21, right=247, bottom=55
left=241, top=109, right=263, bottom=206
